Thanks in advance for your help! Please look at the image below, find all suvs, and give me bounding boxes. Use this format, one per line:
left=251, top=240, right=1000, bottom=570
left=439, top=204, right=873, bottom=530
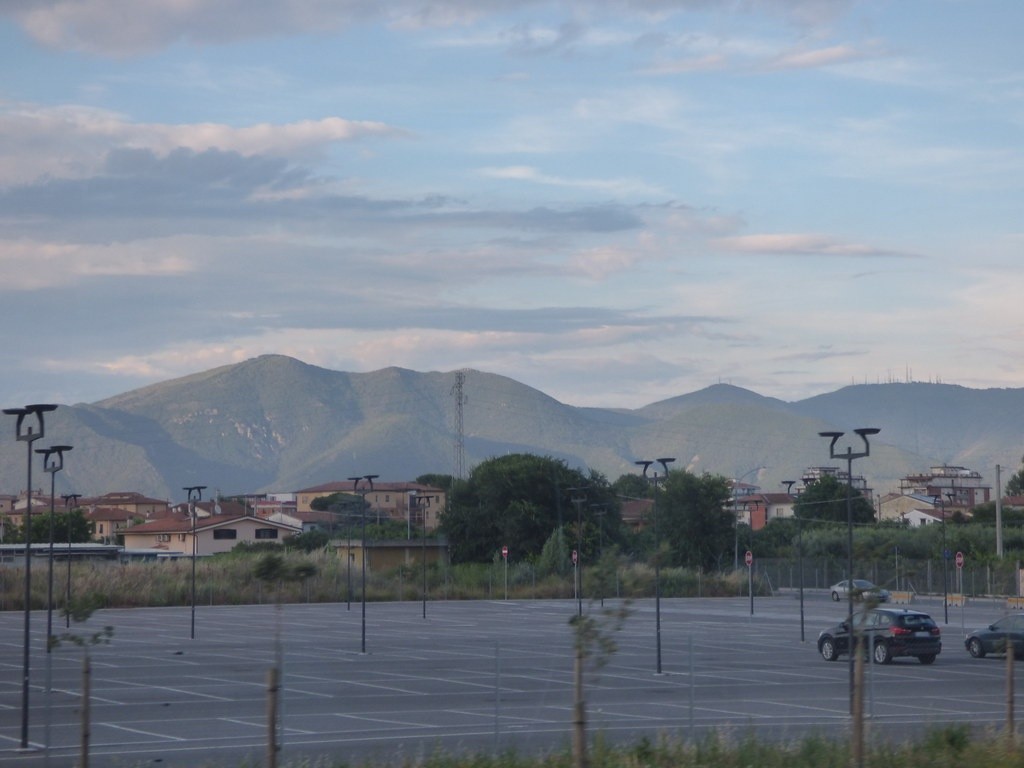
left=816, top=607, right=942, bottom=665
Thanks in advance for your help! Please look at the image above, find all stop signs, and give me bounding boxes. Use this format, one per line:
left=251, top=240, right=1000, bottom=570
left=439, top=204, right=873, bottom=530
left=955, top=552, right=964, bottom=568
left=501, top=546, right=509, bottom=558
left=573, top=551, right=577, bottom=563
left=745, top=551, right=753, bottom=566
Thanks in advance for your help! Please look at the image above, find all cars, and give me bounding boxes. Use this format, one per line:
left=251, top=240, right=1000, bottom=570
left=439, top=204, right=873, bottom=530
left=964, top=611, right=1024, bottom=660
left=830, top=579, right=890, bottom=603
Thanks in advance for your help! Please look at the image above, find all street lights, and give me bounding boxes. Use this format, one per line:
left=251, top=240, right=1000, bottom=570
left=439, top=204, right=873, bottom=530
left=816, top=428, right=885, bottom=715
left=735, top=465, right=766, bottom=572
left=60, top=493, right=83, bottom=627
left=346, top=475, right=378, bottom=654
left=781, top=477, right=816, bottom=643
left=566, top=486, right=598, bottom=620
left=635, top=458, right=676, bottom=672
left=0, top=403, right=61, bottom=748
left=414, top=495, right=435, bottom=618
left=33, top=445, right=75, bottom=653
left=182, top=485, right=207, bottom=639
left=929, top=492, right=958, bottom=623
